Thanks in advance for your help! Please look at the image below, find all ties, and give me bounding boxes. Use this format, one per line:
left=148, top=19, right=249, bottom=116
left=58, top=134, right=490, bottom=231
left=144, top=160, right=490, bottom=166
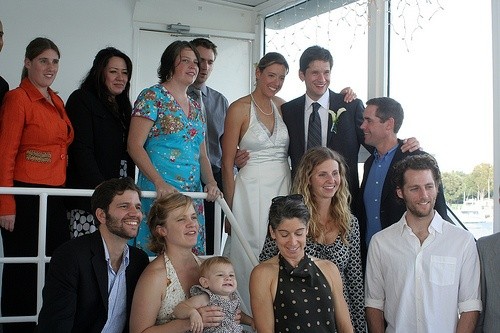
left=306, top=102, right=323, bottom=150
left=193, top=89, right=209, bottom=158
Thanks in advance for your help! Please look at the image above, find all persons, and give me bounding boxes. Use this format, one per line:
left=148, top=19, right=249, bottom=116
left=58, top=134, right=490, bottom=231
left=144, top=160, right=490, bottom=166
left=259, top=146, right=367, bottom=333
left=0, top=20, right=9, bottom=109
left=0, top=38, right=73, bottom=333
left=222, top=52, right=358, bottom=333
left=129, top=194, right=242, bottom=333
left=174, top=256, right=256, bottom=333
left=353, top=97, right=448, bottom=329
left=38, top=179, right=150, bottom=333
left=363, top=155, right=482, bottom=333
left=234, top=45, right=420, bottom=220
left=126, top=40, right=224, bottom=257
left=186, top=38, right=231, bottom=255
left=249, top=194, right=356, bottom=333
left=477, top=186, right=500, bottom=333
left=65, top=47, right=135, bottom=240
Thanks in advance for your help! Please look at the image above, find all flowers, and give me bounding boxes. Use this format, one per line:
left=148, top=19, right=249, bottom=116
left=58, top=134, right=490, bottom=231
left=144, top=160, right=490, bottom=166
left=323, top=107, right=346, bottom=134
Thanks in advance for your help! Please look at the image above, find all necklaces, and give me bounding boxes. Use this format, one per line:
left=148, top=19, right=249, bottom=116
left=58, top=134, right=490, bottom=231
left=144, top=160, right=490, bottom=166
left=250, top=92, right=274, bottom=116
